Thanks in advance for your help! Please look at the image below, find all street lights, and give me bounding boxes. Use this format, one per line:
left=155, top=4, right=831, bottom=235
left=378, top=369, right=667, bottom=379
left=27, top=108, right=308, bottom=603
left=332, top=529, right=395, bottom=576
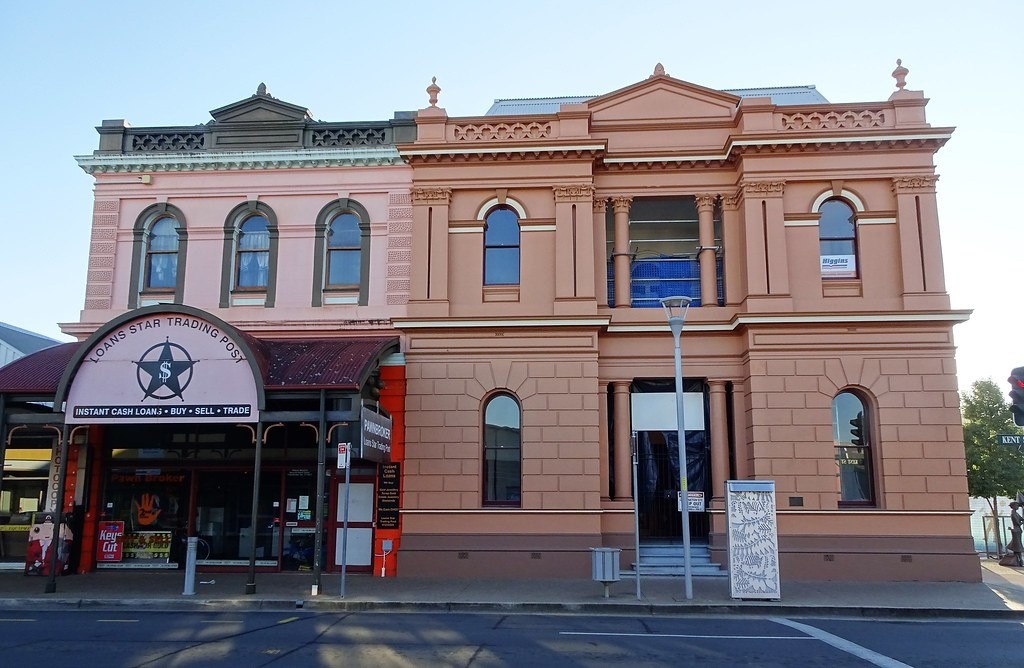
left=659, top=295, right=695, bottom=599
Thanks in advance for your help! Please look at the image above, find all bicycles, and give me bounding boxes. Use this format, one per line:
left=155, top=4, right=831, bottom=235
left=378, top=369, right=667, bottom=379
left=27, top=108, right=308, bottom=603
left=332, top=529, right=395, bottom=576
left=174, top=534, right=211, bottom=563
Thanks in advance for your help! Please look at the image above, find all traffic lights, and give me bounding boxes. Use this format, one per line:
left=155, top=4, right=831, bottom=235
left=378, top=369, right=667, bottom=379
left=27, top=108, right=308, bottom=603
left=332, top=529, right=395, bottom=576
left=850, top=412, right=864, bottom=454
left=1007, top=366, right=1024, bottom=426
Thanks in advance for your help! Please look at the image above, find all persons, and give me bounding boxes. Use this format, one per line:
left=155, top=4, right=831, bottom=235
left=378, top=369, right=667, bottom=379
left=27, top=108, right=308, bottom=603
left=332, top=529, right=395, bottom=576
left=1006, top=502, right=1024, bottom=568
